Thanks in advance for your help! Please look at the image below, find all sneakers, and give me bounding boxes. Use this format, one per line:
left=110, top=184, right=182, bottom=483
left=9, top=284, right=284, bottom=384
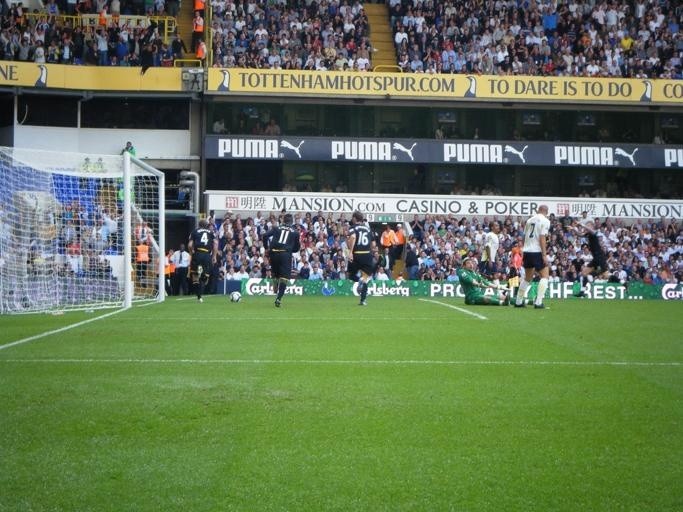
left=272, top=282, right=281, bottom=308
left=573, top=279, right=628, bottom=298
left=356, top=276, right=368, bottom=306
left=505, top=290, right=546, bottom=309
left=194, top=263, right=204, bottom=303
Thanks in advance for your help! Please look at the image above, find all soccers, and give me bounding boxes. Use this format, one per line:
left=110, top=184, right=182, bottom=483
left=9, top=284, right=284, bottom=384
left=229, top=292, right=240, bottom=302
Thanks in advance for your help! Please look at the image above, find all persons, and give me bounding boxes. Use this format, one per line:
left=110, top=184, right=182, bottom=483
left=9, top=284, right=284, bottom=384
left=514, top=205, right=550, bottom=309
left=167, top=249, right=174, bottom=292
left=457, top=259, right=514, bottom=306
left=209, top=102, right=682, bottom=200
left=79, top=156, right=92, bottom=173
left=187, top=220, right=218, bottom=303
left=169, top=243, right=190, bottom=294
left=157, top=255, right=170, bottom=294
left=203, top=202, right=682, bottom=280
left=0, top=202, right=149, bottom=288
left=262, top=214, right=301, bottom=307
left=91, top=158, right=107, bottom=175
left=118, top=187, right=134, bottom=204
left=345, top=211, right=374, bottom=306
left=119, top=141, right=135, bottom=157
left=563, top=214, right=629, bottom=295
left=0, top=0, right=682, bottom=83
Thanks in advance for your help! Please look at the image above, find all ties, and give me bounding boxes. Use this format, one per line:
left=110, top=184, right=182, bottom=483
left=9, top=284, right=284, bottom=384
left=179, top=251, right=182, bottom=264
